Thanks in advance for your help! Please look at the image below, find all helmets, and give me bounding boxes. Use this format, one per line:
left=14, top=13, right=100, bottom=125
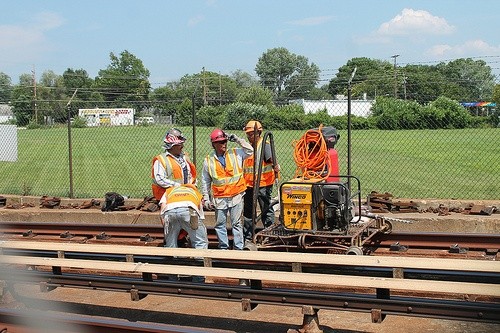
left=245, top=120, right=263, bottom=132
left=211, top=129, right=228, bottom=142
left=163, top=128, right=185, bottom=150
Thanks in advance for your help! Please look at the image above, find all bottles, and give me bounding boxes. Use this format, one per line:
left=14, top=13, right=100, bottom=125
left=191, top=211, right=198, bottom=230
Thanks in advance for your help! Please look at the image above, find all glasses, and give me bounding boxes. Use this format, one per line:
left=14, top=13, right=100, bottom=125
left=219, top=141, right=227, bottom=143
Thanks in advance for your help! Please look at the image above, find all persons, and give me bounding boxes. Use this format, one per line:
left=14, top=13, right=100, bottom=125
left=201, top=129, right=254, bottom=251
left=152, top=127, right=198, bottom=208
left=158, top=183, right=209, bottom=261
left=243, top=120, right=280, bottom=250
